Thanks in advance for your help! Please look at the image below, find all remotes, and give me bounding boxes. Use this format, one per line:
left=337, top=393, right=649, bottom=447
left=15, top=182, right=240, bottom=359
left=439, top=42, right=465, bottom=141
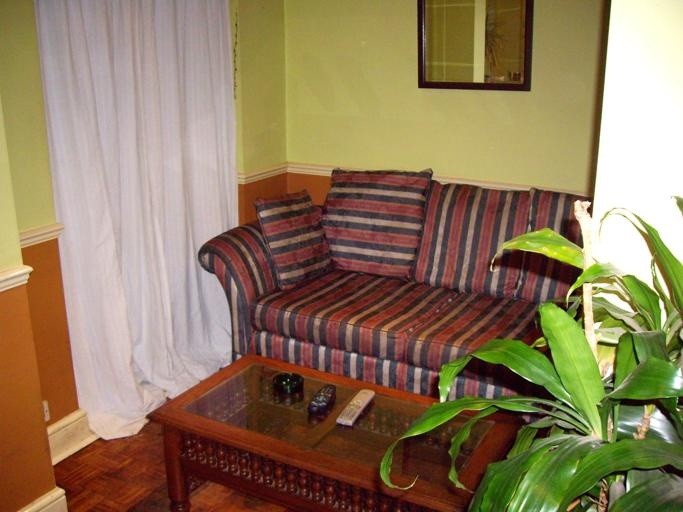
left=308, top=385, right=336, bottom=414
left=335, top=389, right=375, bottom=427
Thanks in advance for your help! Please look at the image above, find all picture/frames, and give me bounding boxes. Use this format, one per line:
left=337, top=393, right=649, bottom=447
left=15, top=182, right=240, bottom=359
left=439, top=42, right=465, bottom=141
left=416, top=0, right=533, bottom=92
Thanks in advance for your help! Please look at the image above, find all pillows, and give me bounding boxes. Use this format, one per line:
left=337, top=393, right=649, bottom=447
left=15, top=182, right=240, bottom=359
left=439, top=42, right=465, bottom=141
left=322, top=168, right=592, bottom=309
left=254, top=187, right=331, bottom=292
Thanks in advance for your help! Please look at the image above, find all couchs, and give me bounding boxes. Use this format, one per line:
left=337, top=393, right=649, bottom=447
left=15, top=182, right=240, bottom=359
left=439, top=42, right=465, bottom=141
left=196, top=203, right=592, bottom=439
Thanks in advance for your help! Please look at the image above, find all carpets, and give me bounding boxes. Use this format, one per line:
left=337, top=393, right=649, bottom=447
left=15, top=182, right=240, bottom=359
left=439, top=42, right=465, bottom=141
left=129, top=477, right=291, bottom=512
